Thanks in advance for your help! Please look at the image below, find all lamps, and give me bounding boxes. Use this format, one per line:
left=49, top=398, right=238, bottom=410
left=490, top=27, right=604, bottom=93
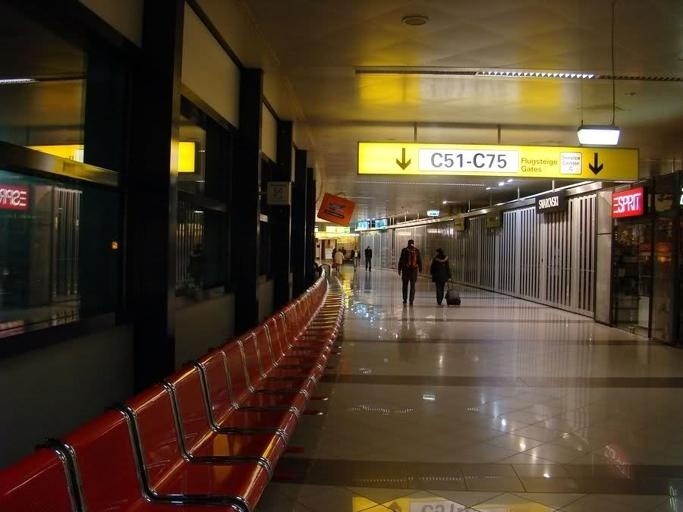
left=576, top=75, right=620, bottom=146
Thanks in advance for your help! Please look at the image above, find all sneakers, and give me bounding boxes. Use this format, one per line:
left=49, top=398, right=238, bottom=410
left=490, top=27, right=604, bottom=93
left=437, top=298, right=443, bottom=305
left=409, top=300, right=413, bottom=305
left=403, top=299, right=407, bottom=304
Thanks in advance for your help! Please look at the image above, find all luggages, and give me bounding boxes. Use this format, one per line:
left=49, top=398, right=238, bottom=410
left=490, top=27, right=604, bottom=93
left=445, top=278, right=461, bottom=306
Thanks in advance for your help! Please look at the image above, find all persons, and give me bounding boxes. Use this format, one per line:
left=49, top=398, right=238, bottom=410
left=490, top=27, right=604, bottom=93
left=398, top=240, right=423, bottom=305
left=429, top=248, right=451, bottom=306
left=364, top=245, right=373, bottom=272
left=332, top=246, right=362, bottom=273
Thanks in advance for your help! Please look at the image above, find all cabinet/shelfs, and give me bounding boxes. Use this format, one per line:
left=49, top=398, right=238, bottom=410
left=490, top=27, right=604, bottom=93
left=611, top=255, right=673, bottom=330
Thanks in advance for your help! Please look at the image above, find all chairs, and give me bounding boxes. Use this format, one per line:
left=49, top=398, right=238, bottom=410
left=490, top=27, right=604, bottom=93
left=236, top=266, right=345, bottom=415
left=2, top=442, right=78, bottom=510
left=118, top=384, right=271, bottom=510
left=199, top=351, right=298, bottom=445
left=47, top=407, right=242, bottom=510
left=219, top=341, right=308, bottom=421
left=160, top=364, right=284, bottom=479
